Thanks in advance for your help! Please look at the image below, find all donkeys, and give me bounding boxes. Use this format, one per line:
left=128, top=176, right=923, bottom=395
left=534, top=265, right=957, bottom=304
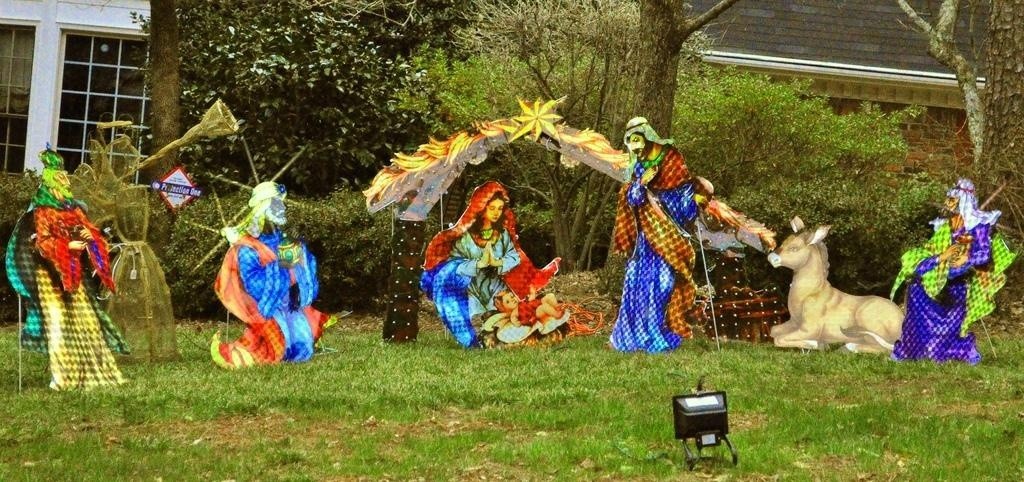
left=765, top=215, right=906, bottom=358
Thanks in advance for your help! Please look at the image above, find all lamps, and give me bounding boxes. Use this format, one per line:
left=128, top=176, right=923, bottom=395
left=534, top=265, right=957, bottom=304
left=672, top=389, right=740, bottom=468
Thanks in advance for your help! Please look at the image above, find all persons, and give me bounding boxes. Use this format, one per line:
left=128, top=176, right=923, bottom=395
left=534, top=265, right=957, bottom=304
left=607, top=114, right=712, bottom=353
left=888, top=174, right=1017, bottom=363
left=493, top=288, right=569, bottom=327
left=0, top=140, right=129, bottom=393
left=206, top=177, right=354, bottom=374
left=420, top=180, right=606, bottom=348
